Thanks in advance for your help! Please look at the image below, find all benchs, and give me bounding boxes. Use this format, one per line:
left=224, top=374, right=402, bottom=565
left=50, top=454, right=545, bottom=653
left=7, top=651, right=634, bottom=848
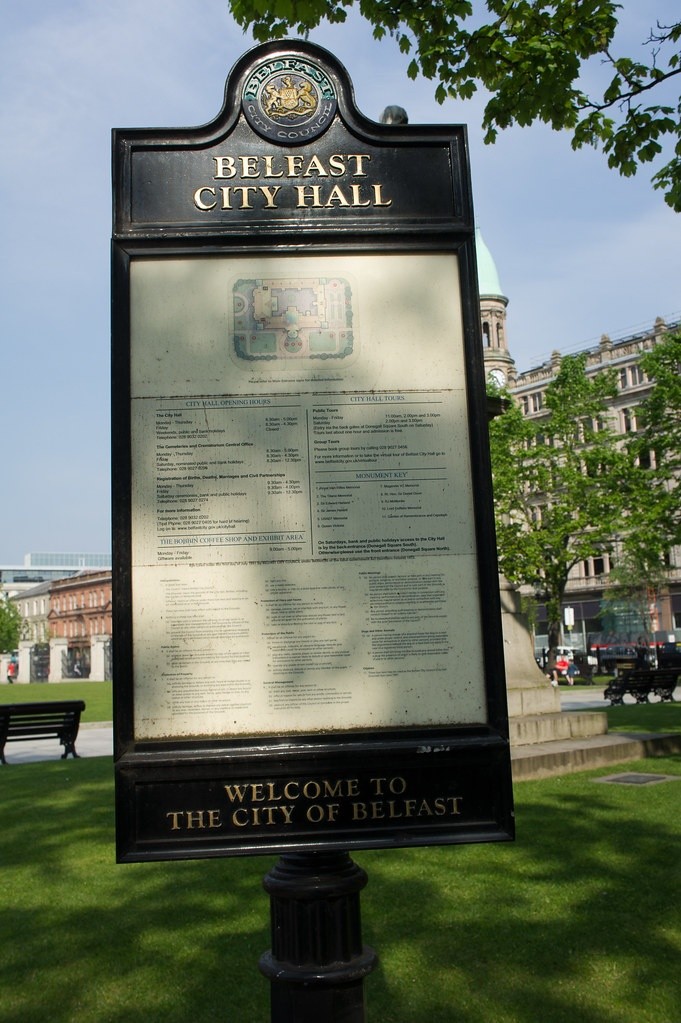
left=550, top=664, right=597, bottom=686
left=0, top=699, right=86, bottom=765
left=604, top=667, right=681, bottom=706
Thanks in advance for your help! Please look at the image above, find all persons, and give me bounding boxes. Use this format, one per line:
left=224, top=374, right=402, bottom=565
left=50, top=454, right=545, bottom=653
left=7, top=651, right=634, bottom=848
left=548, top=655, right=580, bottom=686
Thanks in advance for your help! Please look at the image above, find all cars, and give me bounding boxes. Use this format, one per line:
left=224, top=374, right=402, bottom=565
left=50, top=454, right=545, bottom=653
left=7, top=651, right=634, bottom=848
left=538, top=641, right=681, bottom=672
